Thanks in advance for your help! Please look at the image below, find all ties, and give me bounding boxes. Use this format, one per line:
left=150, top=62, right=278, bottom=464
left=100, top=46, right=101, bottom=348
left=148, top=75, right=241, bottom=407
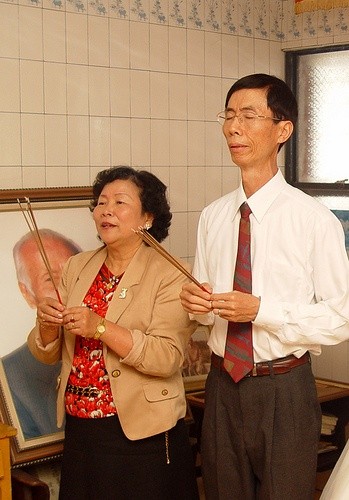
left=224, top=201, right=255, bottom=383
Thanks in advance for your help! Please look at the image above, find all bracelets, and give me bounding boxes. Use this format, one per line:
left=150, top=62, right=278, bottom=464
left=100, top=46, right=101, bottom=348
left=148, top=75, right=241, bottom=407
left=40, top=325, right=57, bottom=332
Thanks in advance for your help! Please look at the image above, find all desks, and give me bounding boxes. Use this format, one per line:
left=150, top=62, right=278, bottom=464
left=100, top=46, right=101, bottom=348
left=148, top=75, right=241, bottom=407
left=184, top=377, right=349, bottom=471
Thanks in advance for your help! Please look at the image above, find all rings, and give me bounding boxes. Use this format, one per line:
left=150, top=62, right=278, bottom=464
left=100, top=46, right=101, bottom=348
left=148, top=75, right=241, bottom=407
left=72, top=322, right=75, bottom=328
left=71, top=314, right=74, bottom=322
left=41, top=313, right=46, bottom=322
left=218, top=309, right=221, bottom=315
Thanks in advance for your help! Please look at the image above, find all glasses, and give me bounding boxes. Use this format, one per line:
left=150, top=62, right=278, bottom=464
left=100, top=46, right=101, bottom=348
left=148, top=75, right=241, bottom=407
left=216, top=110, right=287, bottom=126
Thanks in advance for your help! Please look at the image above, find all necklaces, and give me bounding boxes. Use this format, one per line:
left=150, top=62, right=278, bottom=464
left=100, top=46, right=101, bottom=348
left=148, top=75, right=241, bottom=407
left=106, top=255, right=125, bottom=289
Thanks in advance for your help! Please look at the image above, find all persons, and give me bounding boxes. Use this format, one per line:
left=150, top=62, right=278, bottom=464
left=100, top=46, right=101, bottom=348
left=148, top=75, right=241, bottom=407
left=179, top=74, right=349, bottom=500
left=27, top=166, right=192, bottom=500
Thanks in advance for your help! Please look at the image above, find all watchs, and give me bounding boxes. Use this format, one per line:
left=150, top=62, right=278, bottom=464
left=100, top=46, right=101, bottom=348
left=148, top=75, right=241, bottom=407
left=93, top=317, right=107, bottom=340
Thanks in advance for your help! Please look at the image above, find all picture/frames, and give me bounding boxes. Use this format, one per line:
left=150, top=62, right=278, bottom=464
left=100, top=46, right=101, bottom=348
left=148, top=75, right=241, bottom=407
left=181, top=324, right=215, bottom=391
left=0, top=186, right=107, bottom=468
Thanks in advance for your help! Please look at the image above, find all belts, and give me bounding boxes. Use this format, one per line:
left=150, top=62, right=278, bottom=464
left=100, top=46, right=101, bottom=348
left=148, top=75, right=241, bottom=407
left=211, top=350, right=312, bottom=377
